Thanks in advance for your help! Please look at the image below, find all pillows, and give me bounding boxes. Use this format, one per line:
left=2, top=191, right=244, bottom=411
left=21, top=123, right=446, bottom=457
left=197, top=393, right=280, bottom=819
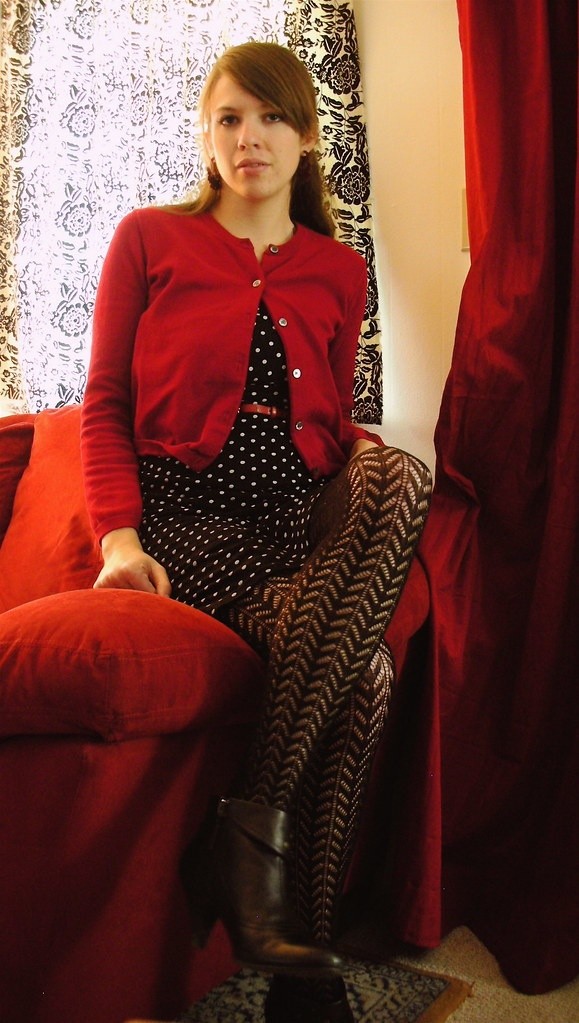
left=1, top=589, right=269, bottom=748
left=0, top=405, right=111, bottom=613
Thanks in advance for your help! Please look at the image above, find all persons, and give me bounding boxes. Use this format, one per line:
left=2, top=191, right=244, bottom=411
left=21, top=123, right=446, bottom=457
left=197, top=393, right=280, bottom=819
left=80, top=43, right=434, bottom=1022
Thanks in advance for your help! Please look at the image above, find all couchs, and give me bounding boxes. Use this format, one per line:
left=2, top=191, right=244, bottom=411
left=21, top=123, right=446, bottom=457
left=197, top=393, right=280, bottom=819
left=0, top=411, right=435, bottom=1023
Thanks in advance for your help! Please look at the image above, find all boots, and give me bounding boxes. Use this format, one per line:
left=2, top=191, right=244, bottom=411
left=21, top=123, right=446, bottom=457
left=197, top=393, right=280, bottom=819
left=262, top=973, right=355, bottom=1023
left=213, top=797, right=342, bottom=979
